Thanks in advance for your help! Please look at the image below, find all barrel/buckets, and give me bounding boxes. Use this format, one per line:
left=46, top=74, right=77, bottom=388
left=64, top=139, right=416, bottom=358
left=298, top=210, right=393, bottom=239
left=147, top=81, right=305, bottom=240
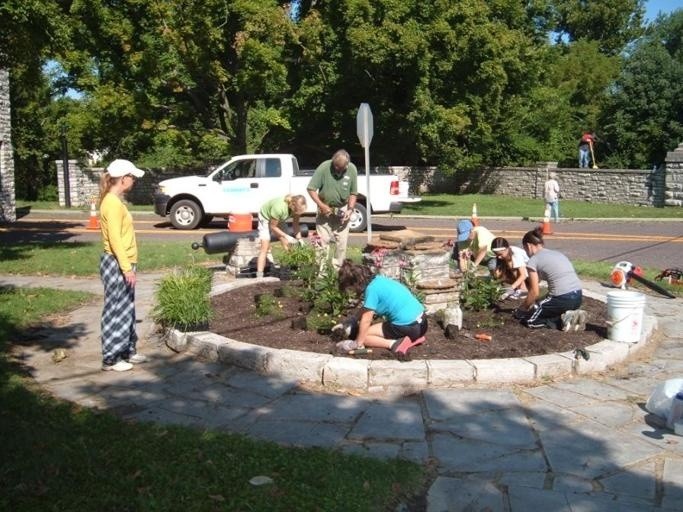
left=604, top=290, right=646, bottom=343
left=227, top=209, right=254, bottom=233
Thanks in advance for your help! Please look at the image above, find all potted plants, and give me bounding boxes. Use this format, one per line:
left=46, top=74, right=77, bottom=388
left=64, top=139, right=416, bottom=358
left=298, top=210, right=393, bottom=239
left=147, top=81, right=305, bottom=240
left=147, top=265, right=215, bottom=333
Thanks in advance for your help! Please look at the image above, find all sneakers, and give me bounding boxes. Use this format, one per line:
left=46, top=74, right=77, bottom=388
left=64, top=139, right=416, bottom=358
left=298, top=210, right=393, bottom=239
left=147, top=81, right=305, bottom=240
left=128, top=354, right=147, bottom=364
left=507, top=288, right=529, bottom=300
left=556, top=309, right=577, bottom=333
left=100, top=359, right=133, bottom=372
left=389, top=334, right=426, bottom=354
left=574, top=308, right=589, bottom=331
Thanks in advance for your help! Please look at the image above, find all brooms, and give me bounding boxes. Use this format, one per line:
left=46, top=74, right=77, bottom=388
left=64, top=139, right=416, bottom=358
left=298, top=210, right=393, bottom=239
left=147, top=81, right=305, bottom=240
left=589, top=140, right=600, bottom=169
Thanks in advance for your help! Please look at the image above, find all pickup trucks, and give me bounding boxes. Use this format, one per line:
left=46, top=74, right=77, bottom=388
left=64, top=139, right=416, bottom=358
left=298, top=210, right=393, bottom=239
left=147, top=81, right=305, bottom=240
left=150, top=150, right=426, bottom=235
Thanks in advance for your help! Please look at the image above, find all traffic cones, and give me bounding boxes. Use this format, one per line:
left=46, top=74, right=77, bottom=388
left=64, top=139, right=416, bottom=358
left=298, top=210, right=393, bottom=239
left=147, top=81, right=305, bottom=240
left=468, top=201, right=480, bottom=227
left=540, top=207, right=554, bottom=234
left=84, top=197, right=101, bottom=231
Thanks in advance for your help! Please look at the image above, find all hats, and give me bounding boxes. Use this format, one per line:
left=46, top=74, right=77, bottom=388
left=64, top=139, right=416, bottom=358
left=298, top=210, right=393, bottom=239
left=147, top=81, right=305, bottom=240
left=454, top=219, right=474, bottom=243
left=105, top=158, right=145, bottom=179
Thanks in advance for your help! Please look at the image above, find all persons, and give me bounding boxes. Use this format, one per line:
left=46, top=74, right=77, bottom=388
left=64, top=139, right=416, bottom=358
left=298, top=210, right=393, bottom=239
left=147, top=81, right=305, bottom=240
left=306, top=149, right=359, bottom=279
left=256, top=194, right=307, bottom=281
left=544, top=172, right=560, bottom=226
left=98, top=158, right=146, bottom=371
left=514, top=227, right=582, bottom=332
left=338, top=259, right=429, bottom=356
left=450, top=220, right=497, bottom=277
left=491, top=236, right=530, bottom=302
left=578, top=133, right=597, bottom=169
